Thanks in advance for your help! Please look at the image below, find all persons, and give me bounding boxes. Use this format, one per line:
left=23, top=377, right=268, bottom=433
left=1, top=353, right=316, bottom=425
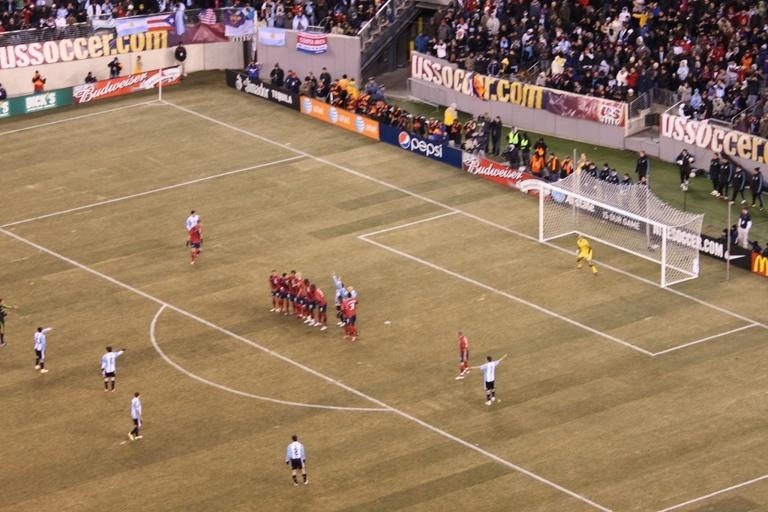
left=128, top=392, right=144, bottom=441
left=285, top=435, right=308, bottom=487
left=455, top=331, right=471, bottom=380
left=33, top=326, right=56, bottom=373
left=101, top=346, right=126, bottom=392
left=469, top=354, right=507, bottom=405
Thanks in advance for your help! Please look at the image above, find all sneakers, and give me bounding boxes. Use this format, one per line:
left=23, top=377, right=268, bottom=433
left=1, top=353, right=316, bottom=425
left=186, top=241, right=201, bottom=266
left=34, top=364, right=49, bottom=374
left=455, top=370, right=470, bottom=381
left=127, top=432, right=143, bottom=442
left=104, top=388, right=115, bottom=394
left=484, top=396, right=496, bottom=406
left=269, top=305, right=328, bottom=331
left=292, top=480, right=309, bottom=488
left=680, top=180, right=689, bottom=191
left=710, top=189, right=764, bottom=212
left=336, top=321, right=359, bottom=343
left=0, top=342, right=7, bottom=349
